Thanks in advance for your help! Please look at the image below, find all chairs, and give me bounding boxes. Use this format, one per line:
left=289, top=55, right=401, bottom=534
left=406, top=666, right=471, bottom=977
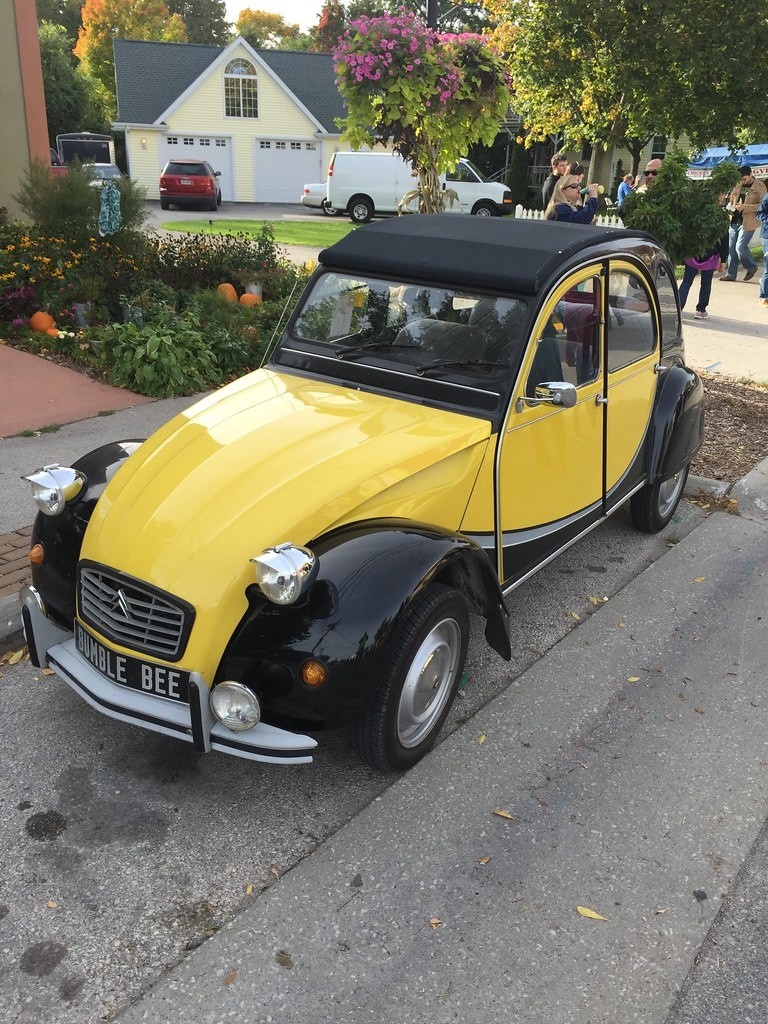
left=392, top=317, right=488, bottom=363
left=493, top=338, right=593, bottom=387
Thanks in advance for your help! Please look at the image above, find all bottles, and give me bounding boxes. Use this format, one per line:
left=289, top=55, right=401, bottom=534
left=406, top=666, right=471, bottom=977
left=579, top=185, right=604, bottom=196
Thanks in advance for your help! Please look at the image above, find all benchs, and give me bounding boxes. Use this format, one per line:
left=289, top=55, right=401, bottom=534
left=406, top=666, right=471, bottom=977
left=468, top=298, right=652, bottom=370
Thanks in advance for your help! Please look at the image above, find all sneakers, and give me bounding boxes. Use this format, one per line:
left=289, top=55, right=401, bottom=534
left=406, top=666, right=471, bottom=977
left=693, top=310, right=710, bottom=319
left=753, top=298, right=768, bottom=308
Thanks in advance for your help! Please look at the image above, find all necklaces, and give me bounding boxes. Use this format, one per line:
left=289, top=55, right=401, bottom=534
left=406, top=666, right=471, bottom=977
left=568, top=200, right=579, bottom=211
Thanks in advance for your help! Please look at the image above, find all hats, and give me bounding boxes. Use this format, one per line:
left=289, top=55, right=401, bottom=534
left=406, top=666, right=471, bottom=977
left=738, top=166, right=752, bottom=175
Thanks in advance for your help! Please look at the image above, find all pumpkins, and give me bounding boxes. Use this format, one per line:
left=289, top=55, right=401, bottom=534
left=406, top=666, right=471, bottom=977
left=46, top=324, right=60, bottom=338
left=239, top=290, right=260, bottom=307
left=30, top=310, right=54, bottom=333
left=216, top=282, right=238, bottom=302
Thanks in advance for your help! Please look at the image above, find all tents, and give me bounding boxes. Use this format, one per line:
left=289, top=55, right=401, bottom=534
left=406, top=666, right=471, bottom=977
left=683, top=143, right=768, bottom=184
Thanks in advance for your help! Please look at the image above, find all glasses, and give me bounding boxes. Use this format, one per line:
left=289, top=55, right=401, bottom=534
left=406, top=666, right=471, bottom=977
left=561, top=183, right=583, bottom=190
left=642, top=170, right=659, bottom=176
left=573, top=160, right=581, bottom=170
left=553, top=155, right=567, bottom=165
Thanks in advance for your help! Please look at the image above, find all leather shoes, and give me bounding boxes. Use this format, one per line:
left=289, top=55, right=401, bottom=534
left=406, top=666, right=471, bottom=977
left=742, top=266, right=758, bottom=281
left=719, top=275, right=736, bottom=281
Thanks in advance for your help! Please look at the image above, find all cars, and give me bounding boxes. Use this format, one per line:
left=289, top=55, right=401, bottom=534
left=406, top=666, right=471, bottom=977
left=18, top=213, right=706, bottom=775
left=50, top=147, right=72, bottom=180
left=82, top=163, right=124, bottom=194
left=159, top=159, right=222, bottom=211
left=301, top=184, right=343, bottom=216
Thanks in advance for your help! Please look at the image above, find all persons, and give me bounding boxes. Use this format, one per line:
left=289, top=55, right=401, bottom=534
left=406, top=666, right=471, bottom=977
left=756, top=193, right=768, bottom=308
left=636, top=159, right=662, bottom=193
left=564, top=161, right=584, bottom=183
left=545, top=176, right=598, bottom=225
left=719, top=165, right=768, bottom=282
left=618, top=173, right=642, bottom=228
left=541, top=154, right=568, bottom=213
left=678, top=195, right=729, bottom=319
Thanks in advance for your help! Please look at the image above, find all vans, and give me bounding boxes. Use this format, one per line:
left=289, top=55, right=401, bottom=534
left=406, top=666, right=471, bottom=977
left=327, top=151, right=513, bottom=223
